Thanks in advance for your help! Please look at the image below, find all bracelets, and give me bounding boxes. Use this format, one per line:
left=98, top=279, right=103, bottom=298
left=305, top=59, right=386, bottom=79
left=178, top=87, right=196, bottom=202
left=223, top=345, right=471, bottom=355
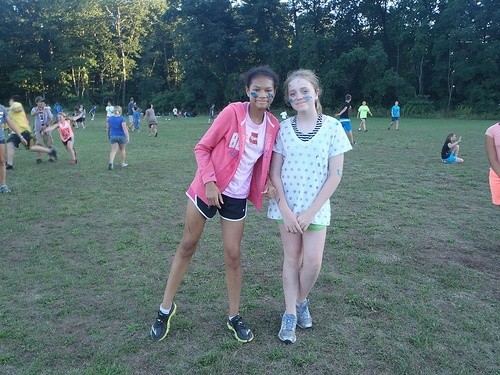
left=19, top=136, right=24, bottom=139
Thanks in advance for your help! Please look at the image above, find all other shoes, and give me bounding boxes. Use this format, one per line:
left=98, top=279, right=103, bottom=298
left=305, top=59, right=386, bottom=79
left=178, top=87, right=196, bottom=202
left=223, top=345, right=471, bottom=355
left=127, top=125, right=131, bottom=132
left=0, top=184, right=11, bottom=193
left=364, top=130, right=368, bottom=132
left=5, top=162, right=13, bottom=170
left=358, top=128, right=361, bottom=131
left=155, top=132, right=158, bottom=137
left=109, top=161, right=113, bottom=169
left=388, top=127, right=390, bottom=130
left=36, top=158, right=42, bottom=163
left=71, top=159, right=77, bottom=164
left=120, top=164, right=128, bottom=167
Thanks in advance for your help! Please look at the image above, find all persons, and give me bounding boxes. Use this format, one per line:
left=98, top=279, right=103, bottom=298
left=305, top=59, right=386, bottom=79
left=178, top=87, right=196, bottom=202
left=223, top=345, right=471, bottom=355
left=208, top=104, right=216, bottom=124
left=0, top=103, right=28, bottom=193
left=172, top=106, right=190, bottom=119
left=270, top=68, right=352, bottom=343
left=279, top=110, right=288, bottom=121
left=334, top=94, right=355, bottom=150
left=54, top=97, right=159, bottom=137
left=151, top=65, right=276, bottom=342
left=387, top=101, right=400, bottom=132
left=5, top=94, right=57, bottom=170
left=31, top=96, right=57, bottom=163
left=440, top=132, right=465, bottom=164
left=43, top=111, right=84, bottom=164
left=356, top=100, right=372, bottom=132
left=42, top=100, right=48, bottom=107
left=485, top=121, right=500, bottom=205
left=229, top=99, right=233, bottom=103
left=106, top=106, right=131, bottom=170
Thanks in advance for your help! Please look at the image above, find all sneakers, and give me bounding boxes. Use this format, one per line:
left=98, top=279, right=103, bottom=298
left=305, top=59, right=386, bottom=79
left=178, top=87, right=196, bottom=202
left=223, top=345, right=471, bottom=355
left=278, top=311, right=297, bottom=344
left=295, top=299, right=313, bottom=329
left=227, top=315, right=253, bottom=342
left=151, top=302, right=177, bottom=341
